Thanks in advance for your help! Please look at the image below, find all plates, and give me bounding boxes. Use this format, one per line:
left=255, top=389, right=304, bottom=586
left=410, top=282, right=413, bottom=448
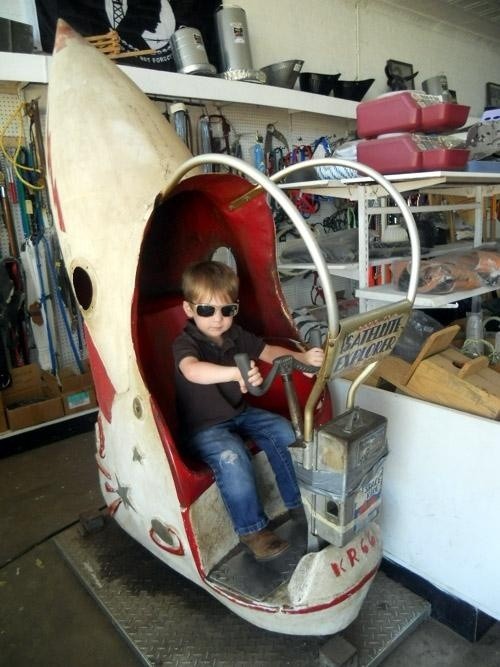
left=341, top=170, right=500, bottom=308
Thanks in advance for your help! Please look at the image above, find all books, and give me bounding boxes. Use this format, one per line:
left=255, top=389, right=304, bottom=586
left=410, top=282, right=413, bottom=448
left=1, top=358, right=99, bottom=433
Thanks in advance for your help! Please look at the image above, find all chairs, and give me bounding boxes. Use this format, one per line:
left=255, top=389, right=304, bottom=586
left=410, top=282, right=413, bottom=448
left=238, top=528, right=289, bottom=561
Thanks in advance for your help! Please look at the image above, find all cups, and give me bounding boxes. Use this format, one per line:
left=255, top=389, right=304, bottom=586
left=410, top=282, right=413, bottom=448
left=188, top=300, right=240, bottom=317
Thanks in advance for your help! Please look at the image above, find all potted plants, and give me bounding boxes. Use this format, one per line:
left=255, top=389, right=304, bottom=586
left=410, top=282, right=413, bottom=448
left=387, top=59, right=415, bottom=91
left=487, top=81, right=500, bottom=108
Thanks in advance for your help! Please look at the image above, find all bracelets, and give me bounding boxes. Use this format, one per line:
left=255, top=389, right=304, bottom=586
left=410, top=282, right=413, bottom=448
left=259, top=59, right=375, bottom=102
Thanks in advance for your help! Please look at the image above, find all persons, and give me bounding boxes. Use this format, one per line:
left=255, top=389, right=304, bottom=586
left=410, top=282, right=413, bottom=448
left=171, top=261, right=326, bottom=562
